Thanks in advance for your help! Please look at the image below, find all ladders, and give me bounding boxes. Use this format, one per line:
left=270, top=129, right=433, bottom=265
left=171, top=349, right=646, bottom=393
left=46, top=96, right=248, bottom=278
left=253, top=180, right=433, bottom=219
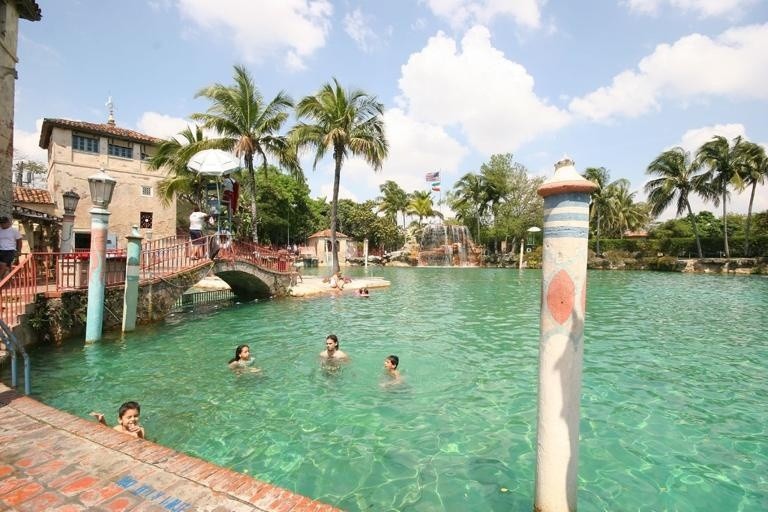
left=216, top=176, right=233, bottom=235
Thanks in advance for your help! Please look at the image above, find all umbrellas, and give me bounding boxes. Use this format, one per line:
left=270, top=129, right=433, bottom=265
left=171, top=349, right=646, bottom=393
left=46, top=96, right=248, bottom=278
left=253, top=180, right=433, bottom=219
left=526, top=226, right=542, bottom=245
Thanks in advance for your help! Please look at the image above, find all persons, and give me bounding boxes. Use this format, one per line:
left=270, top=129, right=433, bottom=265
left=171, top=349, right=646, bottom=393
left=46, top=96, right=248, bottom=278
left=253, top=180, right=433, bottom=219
left=89, top=402, right=144, bottom=439
left=378, top=355, right=401, bottom=387
left=228, top=345, right=262, bottom=376
left=0, top=216, right=22, bottom=276
left=189, top=206, right=208, bottom=260
left=253, top=243, right=303, bottom=271
left=223, top=172, right=236, bottom=206
left=330, top=272, right=368, bottom=298
left=319, top=335, right=350, bottom=378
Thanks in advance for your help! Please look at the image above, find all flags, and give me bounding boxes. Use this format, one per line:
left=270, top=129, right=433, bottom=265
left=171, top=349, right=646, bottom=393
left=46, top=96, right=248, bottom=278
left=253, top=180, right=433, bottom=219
left=426, top=171, right=441, bottom=192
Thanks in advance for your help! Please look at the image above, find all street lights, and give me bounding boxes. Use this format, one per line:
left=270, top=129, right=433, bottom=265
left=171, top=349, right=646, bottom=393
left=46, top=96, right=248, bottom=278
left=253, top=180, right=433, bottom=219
left=60, top=189, right=80, bottom=252
left=84, top=167, right=117, bottom=348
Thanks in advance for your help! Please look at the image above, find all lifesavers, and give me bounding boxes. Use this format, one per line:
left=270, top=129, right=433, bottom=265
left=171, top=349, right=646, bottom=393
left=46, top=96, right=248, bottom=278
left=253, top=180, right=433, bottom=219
left=214, top=230, right=232, bottom=249
